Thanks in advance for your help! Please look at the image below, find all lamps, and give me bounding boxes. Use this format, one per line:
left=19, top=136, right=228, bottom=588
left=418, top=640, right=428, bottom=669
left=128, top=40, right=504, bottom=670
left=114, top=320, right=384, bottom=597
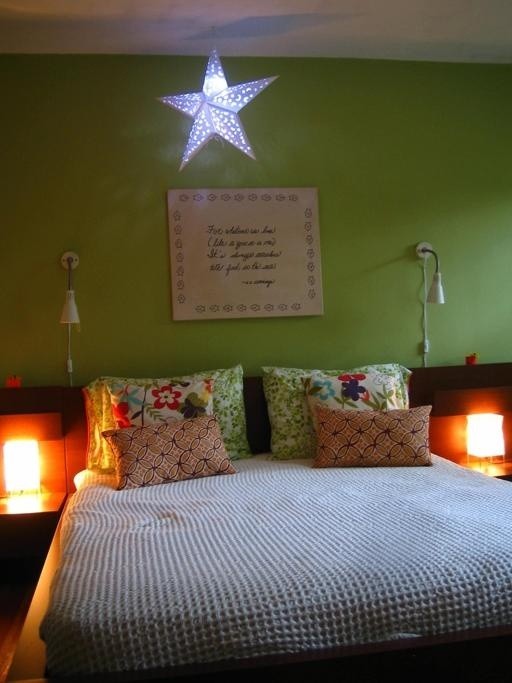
left=59, top=251, right=81, bottom=323
left=414, top=240, right=444, bottom=306
left=156, top=27, right=282, bottom=171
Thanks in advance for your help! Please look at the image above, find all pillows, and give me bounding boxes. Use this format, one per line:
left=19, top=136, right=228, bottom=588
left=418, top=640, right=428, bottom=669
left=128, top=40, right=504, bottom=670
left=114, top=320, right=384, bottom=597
left=98, top=415, right=238, bottom=490
left=105, top=376, right=219, bottom=430
left=259, top=365, right=414, bottom=464
left=310, top=403, right=432, bottom=470
left=299, top=370, right=408, bottom=432
left=85, top=364, right=254, bottom=467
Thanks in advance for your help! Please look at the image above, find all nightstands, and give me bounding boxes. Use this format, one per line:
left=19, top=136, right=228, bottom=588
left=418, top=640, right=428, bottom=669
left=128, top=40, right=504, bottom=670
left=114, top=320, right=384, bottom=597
left=1, top=494, right=66, bottom=582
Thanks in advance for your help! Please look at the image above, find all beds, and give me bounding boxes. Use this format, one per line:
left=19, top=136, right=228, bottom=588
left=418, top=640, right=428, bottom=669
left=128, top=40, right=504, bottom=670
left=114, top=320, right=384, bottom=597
left=45, top=380, right=512, bottom=679
